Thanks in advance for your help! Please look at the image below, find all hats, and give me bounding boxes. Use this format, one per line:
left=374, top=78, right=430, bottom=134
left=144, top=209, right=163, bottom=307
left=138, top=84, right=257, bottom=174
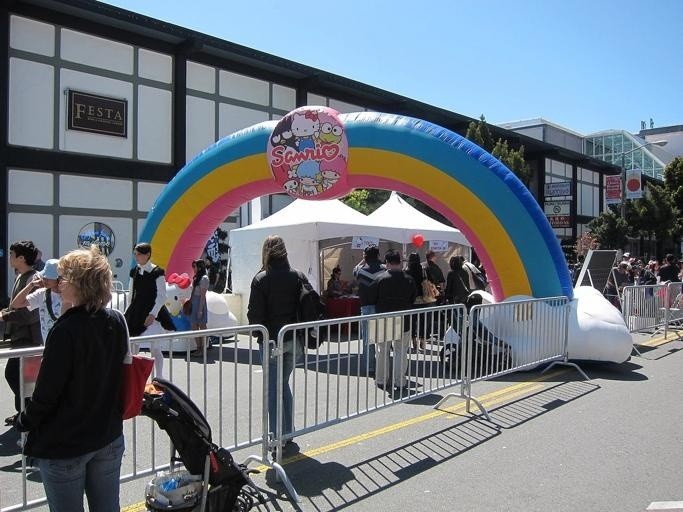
left=623, top=252, right=631, bottom=258
left=38, top=259, right=60, bottom=280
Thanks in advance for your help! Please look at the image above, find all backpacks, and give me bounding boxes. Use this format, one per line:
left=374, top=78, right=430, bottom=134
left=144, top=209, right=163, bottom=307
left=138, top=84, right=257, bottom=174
left=294, top=268, right=328, bottom=349
left=465, top=264, right=488, bottom=291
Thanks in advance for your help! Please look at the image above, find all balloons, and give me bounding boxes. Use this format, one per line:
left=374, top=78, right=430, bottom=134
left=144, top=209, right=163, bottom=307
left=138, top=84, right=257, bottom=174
left=413, top=234, right=425, bottom=248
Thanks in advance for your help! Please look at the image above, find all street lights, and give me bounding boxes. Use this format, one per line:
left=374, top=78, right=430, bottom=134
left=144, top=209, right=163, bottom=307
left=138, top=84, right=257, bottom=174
left=620, top=139, right=670, bottom=220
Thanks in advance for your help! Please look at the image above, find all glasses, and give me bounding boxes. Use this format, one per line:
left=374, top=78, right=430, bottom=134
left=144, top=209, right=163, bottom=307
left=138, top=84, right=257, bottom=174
left=56, top=275, right=70, bottom=284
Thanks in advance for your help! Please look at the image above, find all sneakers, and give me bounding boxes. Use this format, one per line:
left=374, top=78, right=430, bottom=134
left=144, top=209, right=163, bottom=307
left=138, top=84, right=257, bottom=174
left=375, top=381, right=407, bottom=391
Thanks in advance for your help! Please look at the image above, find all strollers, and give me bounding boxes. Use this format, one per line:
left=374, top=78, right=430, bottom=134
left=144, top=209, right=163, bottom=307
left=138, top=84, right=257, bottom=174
left=139, top=376, right=266, bottom=512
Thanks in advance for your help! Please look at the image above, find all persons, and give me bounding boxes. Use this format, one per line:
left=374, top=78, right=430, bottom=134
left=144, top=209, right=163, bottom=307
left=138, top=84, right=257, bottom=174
left=574, top=253, right=683, bottom=316
left=13, top=245, right=128, bottom=512
left=0, top=241, right=210, bottom=425
left=328, top=244, right=486, bottom=390
left=248, top=235, right=308, bottom=451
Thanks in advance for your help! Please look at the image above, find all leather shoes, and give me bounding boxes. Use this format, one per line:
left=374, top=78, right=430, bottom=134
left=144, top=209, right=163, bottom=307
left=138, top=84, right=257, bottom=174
left=192, top=349, right=204, bottom=357
left=5, top=413, right=19, bottom=425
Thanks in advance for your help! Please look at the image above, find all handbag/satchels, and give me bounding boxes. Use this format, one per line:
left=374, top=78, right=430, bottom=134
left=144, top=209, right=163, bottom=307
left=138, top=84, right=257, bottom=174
left=112, top=308, right=155, bottom=420
left=183, top=299, right=193, bottom=315
left=421, top=265, right=440, bottom=303
